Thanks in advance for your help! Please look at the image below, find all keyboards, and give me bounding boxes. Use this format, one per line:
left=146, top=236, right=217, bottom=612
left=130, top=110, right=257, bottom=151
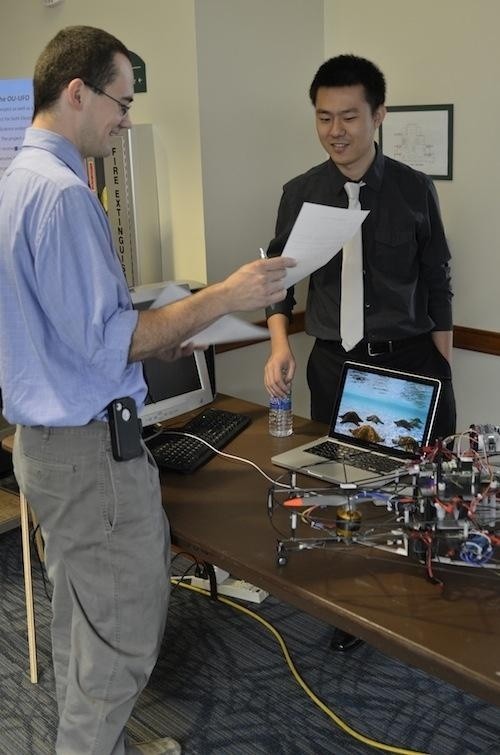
left=149, top=407, right=252, bottom=474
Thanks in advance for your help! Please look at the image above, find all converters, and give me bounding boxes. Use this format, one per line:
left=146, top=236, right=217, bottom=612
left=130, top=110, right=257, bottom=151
left=212, top=566, right=230, bottom=585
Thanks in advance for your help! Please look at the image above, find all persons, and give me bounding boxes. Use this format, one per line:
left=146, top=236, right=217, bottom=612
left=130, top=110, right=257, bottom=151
left=0, top=26, right=297, bottom=755
left=264, top=55, right=456, bottom=655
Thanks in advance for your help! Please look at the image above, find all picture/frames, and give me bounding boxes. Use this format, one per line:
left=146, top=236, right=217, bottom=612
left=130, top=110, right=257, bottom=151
left=377, top=104, right=454, bottom=179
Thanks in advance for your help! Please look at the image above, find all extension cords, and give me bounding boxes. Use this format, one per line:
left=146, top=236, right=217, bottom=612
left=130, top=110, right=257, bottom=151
left=189, top=570, right=268, bottom=604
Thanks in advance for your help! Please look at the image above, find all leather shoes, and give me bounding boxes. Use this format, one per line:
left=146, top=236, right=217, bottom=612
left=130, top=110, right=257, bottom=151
left=329, top=626, right=366, bottom=656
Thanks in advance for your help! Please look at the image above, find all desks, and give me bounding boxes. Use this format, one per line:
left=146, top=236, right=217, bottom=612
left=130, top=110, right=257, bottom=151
left=0, top=392, right=499, bottom=708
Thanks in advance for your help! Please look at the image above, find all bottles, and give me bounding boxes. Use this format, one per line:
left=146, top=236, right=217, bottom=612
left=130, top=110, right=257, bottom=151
left=268, top=367, right=293, bottom=437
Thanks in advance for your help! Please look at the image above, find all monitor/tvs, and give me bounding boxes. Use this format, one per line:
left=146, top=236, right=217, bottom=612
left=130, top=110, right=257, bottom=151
left=130, top=283, right=215, bottom=449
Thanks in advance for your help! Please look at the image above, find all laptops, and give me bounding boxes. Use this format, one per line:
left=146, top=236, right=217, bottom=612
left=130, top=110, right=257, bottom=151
left=271, top=360, right=442, bottom=492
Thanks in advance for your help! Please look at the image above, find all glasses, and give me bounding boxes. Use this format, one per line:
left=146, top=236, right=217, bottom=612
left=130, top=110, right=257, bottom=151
left=68, top=75, right=130, bottom=116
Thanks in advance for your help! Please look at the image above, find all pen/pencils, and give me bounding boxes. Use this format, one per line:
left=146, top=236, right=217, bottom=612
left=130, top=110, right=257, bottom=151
left=259, top=247, right=265, bottom=260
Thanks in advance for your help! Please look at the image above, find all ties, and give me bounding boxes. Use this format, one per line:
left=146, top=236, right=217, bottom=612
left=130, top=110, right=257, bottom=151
left=339, top=180, right=366, bottom=352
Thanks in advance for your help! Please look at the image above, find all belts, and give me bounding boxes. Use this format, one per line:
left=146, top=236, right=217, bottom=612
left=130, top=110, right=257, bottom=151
left=317, top=331, right=434, bottom=358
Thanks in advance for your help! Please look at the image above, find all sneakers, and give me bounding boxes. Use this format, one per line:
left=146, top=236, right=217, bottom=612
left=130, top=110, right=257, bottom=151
left=125, top=736, right=181, bottom=755
